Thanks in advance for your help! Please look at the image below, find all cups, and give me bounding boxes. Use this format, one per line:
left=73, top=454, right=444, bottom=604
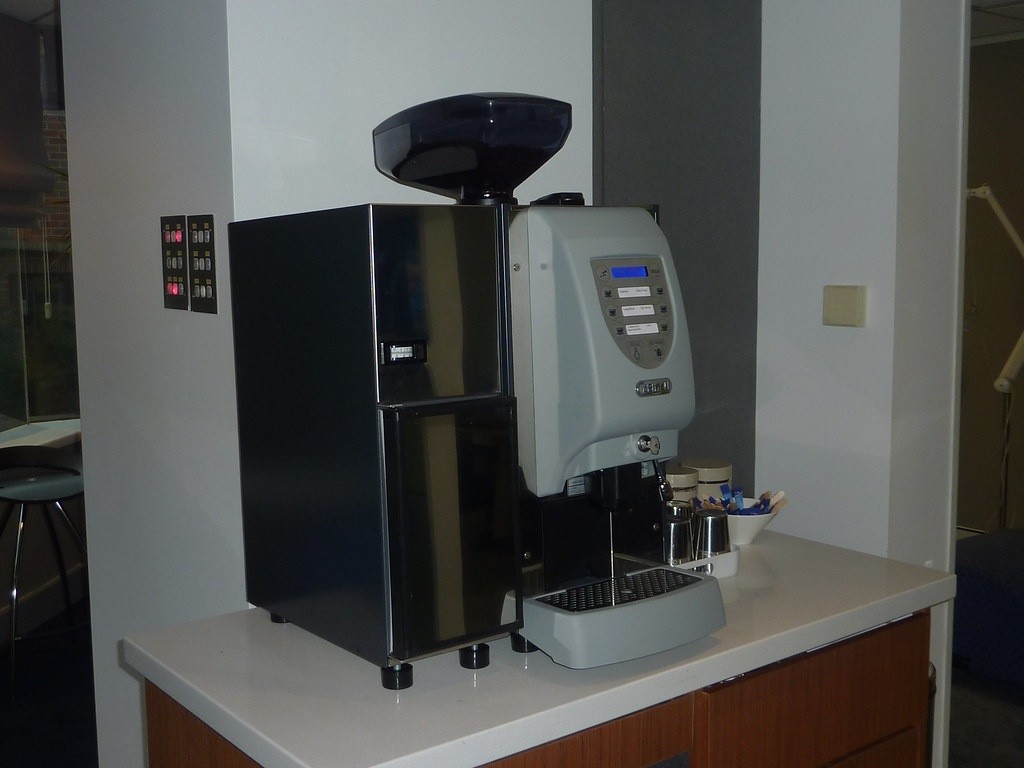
left=665, top=500, right=731, bottom=575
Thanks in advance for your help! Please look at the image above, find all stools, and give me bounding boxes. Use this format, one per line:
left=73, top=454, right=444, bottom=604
left=956, top=524, right=1024, bottom=693
left=0, top=465, right=88, bottom=705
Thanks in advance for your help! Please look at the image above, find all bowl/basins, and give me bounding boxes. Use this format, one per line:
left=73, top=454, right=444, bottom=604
left=726, top=490, right=785, bottom=545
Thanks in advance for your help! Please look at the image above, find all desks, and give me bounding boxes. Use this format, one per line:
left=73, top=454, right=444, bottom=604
left=0, top=418, right=82, bottom=449
left=118, top=528, right=958, bottom=768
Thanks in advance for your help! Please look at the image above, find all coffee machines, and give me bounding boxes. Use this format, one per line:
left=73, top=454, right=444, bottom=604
left=229, top=92, right=727, bottom=691
